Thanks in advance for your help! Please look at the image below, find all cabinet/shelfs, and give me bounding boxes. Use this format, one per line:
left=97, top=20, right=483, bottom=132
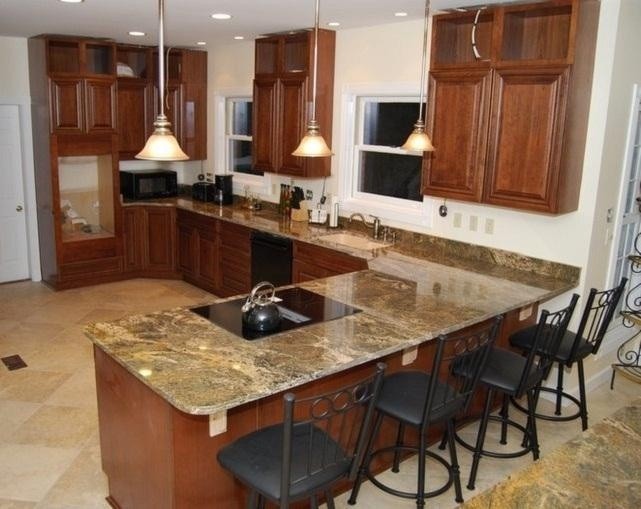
left=180, top=210, right=222, bottom=293
left=414, top=0, right=607, bottom=219
left=122, top=203, right=179, bottom=279
left=28, top=33, right=118, bottom=137
left=117, top=43, right=208, bottom=163
left=32, top=137, right=123, bottom=292
left=222, top=221, right=252, bottom=298
left=293, top=241, right=368, bottom=284
left=248, top=28, right=340, bottom=178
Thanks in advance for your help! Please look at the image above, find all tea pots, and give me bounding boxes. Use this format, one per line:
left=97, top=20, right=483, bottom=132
left=241, top=281, right=281, bottom=331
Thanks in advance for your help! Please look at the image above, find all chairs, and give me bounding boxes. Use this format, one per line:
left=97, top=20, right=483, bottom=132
left=438, top=289, right=581, bottom=492
left=216, top=361, right=388, bottom=509
left=347, top=315, right=506, bottom=507
left=508, top=275, right=633, bottom=436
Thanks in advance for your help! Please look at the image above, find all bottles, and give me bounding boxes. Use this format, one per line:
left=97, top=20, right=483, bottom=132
left=331, top=196, right=339, bottom=227
left=279, top=184, right=291, bottom=214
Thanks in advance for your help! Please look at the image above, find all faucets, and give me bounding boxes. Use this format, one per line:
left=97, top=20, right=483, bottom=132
left=347, top=213, right=381, bottom=239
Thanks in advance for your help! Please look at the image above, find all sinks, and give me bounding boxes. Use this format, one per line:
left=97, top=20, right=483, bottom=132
left=318, top=231, right=393, bottom=250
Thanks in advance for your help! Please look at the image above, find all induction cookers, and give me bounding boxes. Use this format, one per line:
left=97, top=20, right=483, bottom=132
left=188, top=286, right=364, bottom=341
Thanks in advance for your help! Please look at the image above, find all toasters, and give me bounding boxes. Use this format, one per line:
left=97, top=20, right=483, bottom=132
left=310, top=203, right=328, bottom=224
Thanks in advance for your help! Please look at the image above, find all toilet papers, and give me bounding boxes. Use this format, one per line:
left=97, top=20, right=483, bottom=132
left=328, top=195, right=338, bottom=227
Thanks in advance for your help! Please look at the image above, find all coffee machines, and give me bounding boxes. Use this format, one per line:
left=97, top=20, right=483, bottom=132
left=214, top=174, right=233, bottom=204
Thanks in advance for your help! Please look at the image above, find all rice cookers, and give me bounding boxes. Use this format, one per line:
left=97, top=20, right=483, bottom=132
left=193, top=182, right=215, bottom=202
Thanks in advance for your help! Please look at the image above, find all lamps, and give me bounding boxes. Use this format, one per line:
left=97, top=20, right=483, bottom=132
left=291, top=0, right=338, bottom=161
left=132, top=0, right=194, bottom=164
left=402, top=1, right=439, bottom=153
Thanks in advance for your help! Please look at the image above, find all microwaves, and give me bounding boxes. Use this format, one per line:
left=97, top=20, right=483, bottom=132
left=121, top=168, right=177, bottom=201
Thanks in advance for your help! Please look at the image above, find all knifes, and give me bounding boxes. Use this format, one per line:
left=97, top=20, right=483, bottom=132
left=290, top=186, right=305, bottom=209
